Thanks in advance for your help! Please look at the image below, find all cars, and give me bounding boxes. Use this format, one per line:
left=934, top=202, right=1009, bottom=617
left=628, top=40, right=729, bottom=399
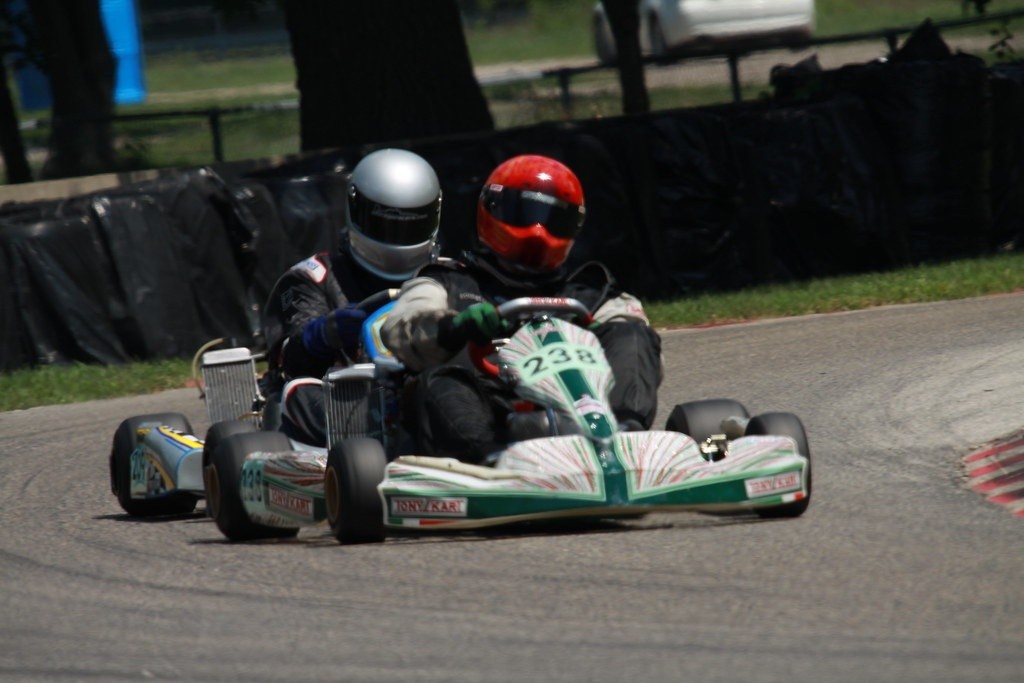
left=590, top=1, right=815, bottom=67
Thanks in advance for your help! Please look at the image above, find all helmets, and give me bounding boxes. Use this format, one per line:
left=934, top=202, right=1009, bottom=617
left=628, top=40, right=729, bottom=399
left=477, top=154, right=585, bottom=268
left=345, top=147, right=443, bottom=280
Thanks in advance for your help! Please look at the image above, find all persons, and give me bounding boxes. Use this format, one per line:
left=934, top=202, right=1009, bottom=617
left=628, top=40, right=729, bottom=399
left=258, top=145, right=474, bottom=444
left=382, top=153, right=664, bottom=465
